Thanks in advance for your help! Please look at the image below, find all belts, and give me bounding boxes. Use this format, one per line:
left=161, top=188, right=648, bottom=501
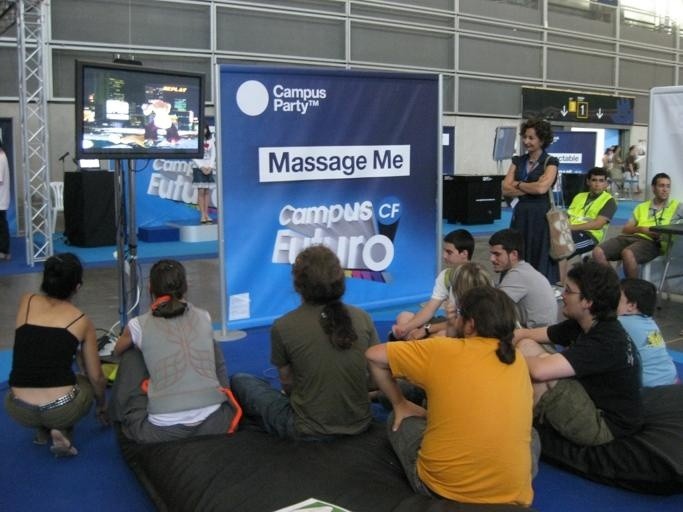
left=9, top=383, right=82, bottom=413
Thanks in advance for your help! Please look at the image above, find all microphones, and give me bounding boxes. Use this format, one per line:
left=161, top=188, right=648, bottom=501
left=59, top=152, right=68, bottom=160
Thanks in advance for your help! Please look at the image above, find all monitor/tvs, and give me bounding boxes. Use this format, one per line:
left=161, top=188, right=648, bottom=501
left=493, top=127, right=517, bottom=159
left=74, top=57, right=204, bottom=159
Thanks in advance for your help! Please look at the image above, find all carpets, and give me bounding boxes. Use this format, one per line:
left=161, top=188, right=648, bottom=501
left=0, top=320, right=681, bottom=512
left=442, top=199, right=647, bottom=239
left=0, top=235, right=45, bottom=276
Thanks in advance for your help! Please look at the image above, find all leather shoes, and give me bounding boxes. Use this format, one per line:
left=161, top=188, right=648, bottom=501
left=200, top=216, right=213, bottom=224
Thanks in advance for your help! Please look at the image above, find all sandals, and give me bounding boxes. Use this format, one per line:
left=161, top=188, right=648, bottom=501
left=51, top=443, right=75, bottom=459
left=32, top=431, right=51, bottom=445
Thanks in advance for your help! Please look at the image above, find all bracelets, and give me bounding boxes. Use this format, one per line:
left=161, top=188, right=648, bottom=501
left=517, top=181, right=522, bottom=187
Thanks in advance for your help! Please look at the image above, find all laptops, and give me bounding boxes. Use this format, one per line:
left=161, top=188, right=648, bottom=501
left=79, top=159, right=109, bottom=171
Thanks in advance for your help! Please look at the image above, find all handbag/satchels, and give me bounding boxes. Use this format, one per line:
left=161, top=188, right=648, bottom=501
left=546, top=203, right=577, bottom=261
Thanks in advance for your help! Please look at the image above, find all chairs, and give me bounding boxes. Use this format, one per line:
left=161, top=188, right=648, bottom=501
left=576, top=222, right=608, bottom=264
left=614, top=237, right=673, bottom=281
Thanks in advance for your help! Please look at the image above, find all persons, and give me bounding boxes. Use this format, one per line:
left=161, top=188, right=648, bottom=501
left=365, top=229, right=678, bottom=506
left=114, top=258, right=242, bottom=444
left=592, top=173, right=683, bottom=280
left=0, top=138, right=11, bottom=258
left=601, top=143, right=642, bottom=199
left=559, top=167, right=618, bottom=285
left=502, top=119, right=559, bottom=285
left=232, top=243, right=381, bottom=443
left=144, top=101, right=178, bottom=142
left=2, top=251, right=106, bottom=458
left=193, top=125, right=217, bottom=222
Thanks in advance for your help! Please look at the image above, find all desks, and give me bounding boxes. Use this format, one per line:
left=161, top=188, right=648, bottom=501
left=648, top=224, right=683, bottom=310
